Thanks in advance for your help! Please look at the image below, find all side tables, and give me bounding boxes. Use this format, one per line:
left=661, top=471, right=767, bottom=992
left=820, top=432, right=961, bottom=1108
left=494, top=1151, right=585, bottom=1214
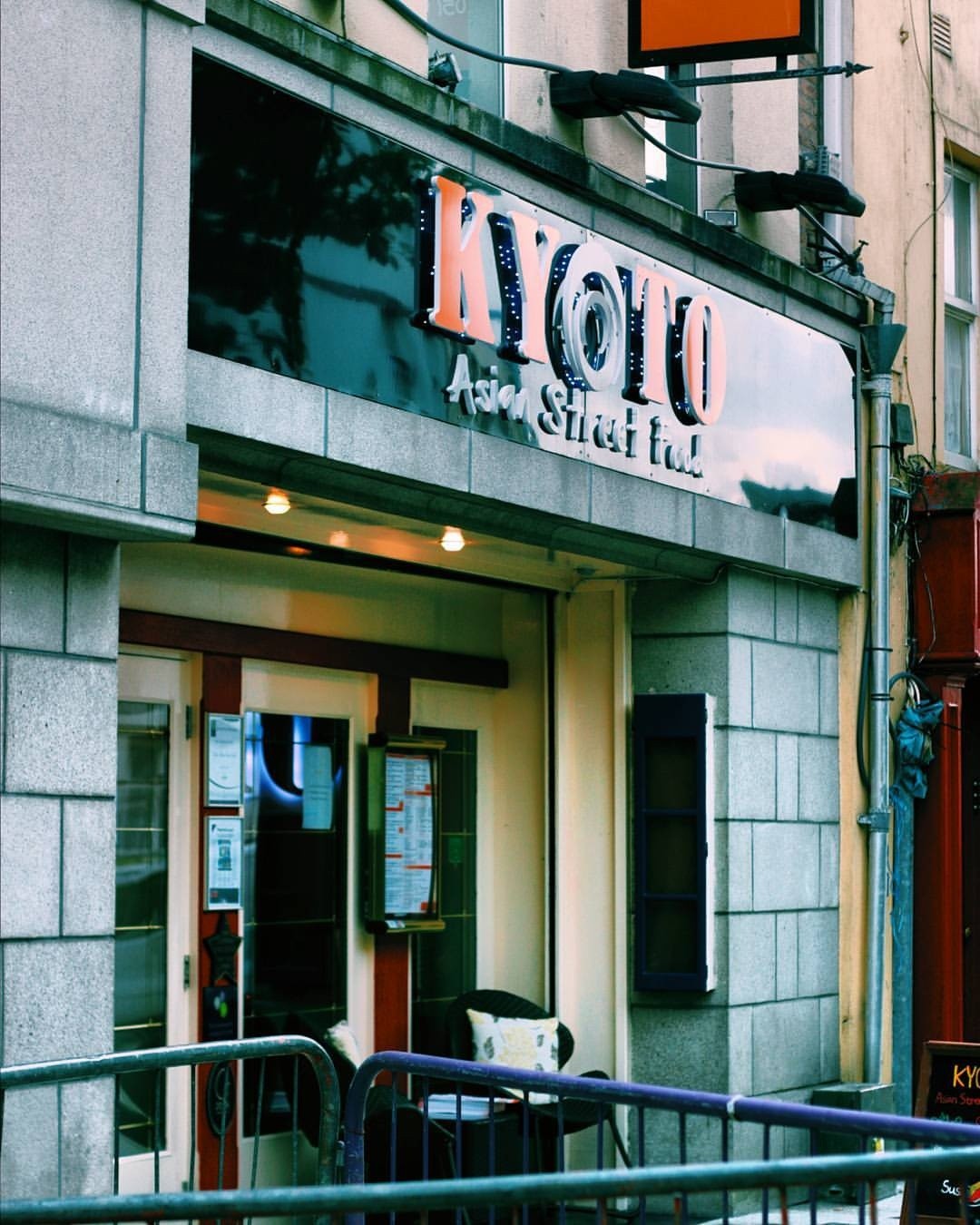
left=405, top=1080, right=547, bottom=1225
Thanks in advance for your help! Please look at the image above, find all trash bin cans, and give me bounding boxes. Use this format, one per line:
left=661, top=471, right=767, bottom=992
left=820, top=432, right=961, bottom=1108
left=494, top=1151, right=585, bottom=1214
left=810, top=1082, right=896, bottom=1206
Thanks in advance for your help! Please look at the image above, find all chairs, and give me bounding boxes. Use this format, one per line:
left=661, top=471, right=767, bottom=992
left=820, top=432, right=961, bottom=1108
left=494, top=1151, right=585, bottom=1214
left=283, top=1011, right=474, bottom=1225
left=422, top=987, right=647, bottom=1225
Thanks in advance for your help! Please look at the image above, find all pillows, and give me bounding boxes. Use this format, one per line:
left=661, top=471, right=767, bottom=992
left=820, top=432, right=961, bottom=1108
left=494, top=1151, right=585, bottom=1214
left=465, top=1008, right=561, bottom=1106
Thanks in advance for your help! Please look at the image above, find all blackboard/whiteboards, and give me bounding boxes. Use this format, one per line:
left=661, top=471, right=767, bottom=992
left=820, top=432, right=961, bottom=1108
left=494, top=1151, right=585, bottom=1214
left=897, top=1040, right=980, bottom=1225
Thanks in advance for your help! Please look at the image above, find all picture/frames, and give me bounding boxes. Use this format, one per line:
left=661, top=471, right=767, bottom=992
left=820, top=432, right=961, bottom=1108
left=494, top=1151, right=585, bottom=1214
left=898, top=1040, right=980, bottom=1224
left=202, top=815, right=243, bottom=913
left=204, top=711, right=245, bottom=809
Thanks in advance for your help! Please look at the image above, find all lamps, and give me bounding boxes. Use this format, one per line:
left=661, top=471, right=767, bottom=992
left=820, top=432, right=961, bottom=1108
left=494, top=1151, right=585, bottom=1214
left=549, top=69, right=701, bottom=124
left=735, top=169, right=866, bottom=218
